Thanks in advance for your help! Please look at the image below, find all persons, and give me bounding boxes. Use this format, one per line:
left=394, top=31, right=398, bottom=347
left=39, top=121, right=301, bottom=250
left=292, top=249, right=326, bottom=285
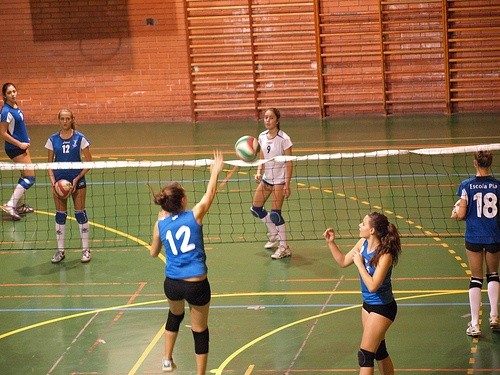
left=0, top=83, right=35, bottom=221
left=322, top=209, right=401, bottom=375
left=151, top=149, right=224, bottom=375
left=250, top=108, right=294, bottom=260
left=450, top=149, right=500, bottom=336
left=46, top=110, right=92, bottom=262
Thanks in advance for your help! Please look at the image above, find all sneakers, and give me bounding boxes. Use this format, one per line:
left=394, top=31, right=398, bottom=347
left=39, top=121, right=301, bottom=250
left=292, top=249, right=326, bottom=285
left=17, top=204, right=34, bottom=214
left=264, top=234, right=280, bottom=248
left=80, top=249, right=91, bottom=262
left=271, top=246, right=291, bottom=259
left=162, top=359, right=176, bottom=371
left=489, top=318, right=500, bottom=331
left=51, top=252, right=65, bottom=263
left=465, top=322, right=481, bottom=336
left=0, top=204, right=20, bottom=220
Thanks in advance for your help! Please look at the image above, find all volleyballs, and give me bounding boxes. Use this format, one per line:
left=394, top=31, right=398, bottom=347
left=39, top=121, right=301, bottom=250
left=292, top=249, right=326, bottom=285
left=53, top=179, right=74, bottom=199
left=234, top=135, right=260, bottom=161
